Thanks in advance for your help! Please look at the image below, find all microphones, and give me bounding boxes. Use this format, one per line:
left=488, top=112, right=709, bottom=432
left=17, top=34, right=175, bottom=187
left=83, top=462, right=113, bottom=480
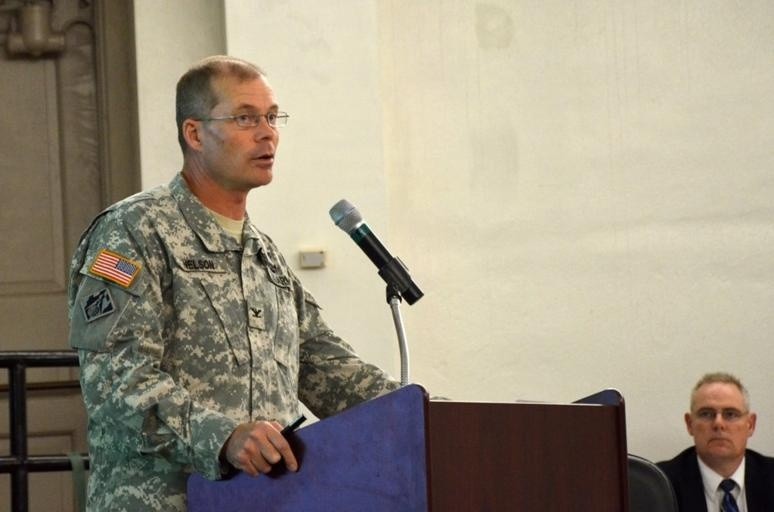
left=329, top=198, right=424, bottom=309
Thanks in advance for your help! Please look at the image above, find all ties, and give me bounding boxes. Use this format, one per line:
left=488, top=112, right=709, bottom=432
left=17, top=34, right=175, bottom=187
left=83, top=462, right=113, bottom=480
left=718, top=478, right=741, bottom=511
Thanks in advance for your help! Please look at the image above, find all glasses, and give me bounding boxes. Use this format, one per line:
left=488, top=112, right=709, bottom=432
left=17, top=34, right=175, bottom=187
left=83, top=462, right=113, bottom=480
left=189, top=109, right=291, bottom=131
left=692, top=407, right=751, bottom=423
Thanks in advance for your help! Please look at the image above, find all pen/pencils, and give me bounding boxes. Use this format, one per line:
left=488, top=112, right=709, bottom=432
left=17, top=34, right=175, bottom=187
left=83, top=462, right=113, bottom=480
left=279, top=416, right=307, bottom=438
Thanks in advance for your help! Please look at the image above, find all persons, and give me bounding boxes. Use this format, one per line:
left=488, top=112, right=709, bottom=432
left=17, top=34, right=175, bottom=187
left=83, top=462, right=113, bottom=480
left=654, top=373, right=774, bottom=512
left=65, top=54, right=452, bottom=512
